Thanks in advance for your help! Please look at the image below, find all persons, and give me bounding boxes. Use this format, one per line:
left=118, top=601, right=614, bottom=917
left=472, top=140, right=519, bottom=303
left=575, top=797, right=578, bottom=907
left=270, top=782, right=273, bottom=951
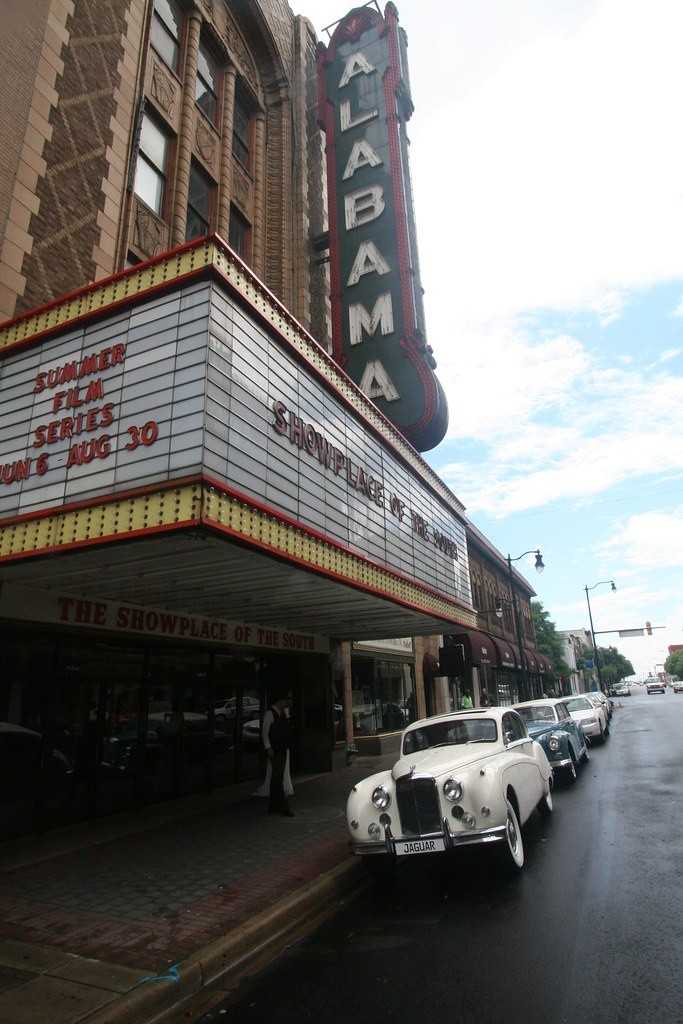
left=547, top=685, right=554, bottom=698
left=480, top=687, right=495, bottom=707
left=406, top=693, right=414, bottom=720
left=262, top=692, right=295, bottom=818
left=460, top=689, right=474, bottom=709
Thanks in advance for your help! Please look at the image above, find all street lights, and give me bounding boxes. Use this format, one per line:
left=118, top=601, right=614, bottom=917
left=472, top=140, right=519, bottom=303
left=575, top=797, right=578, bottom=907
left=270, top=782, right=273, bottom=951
left=504, top=549, right=545, bottom=701
left=584, top=580, right=617, bottom=694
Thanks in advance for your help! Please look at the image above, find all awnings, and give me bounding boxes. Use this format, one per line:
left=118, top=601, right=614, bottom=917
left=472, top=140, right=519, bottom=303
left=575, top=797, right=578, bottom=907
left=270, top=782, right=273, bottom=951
left=423, top=652, right=444, bottom=678
left=442, top=631, right=553, bottom=675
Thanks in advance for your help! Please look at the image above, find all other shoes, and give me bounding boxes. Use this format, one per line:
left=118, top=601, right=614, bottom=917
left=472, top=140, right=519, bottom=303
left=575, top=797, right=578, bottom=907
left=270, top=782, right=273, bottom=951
left=282, top=810, right=295, bottom=818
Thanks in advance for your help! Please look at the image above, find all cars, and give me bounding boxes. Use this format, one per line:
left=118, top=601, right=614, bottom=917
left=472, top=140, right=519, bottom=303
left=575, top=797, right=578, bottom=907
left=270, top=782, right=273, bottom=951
left=508, top=698, right=589, bottom=783
left=673, top=681, right=683, bottom=693
left=204, top=696, right=260, bottom=723
left=615, top=685, right=630, bottom=696
left=346, top=706, right=554, bottom=878
left=398, top=700, right=410, bottom=721
left=559, top=696, right=609, bottom=745
left=90, top=705, right=136, bottom=732
left=353, top=702, right=404, bottom=731
left=582, top=691, right=612, bottom=724
left=242, top=704, right=344, bottom=747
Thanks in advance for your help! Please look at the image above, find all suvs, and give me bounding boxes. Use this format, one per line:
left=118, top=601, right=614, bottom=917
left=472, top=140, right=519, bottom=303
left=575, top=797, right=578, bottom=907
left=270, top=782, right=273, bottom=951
left=646, top=677, right=666, bottom=695
